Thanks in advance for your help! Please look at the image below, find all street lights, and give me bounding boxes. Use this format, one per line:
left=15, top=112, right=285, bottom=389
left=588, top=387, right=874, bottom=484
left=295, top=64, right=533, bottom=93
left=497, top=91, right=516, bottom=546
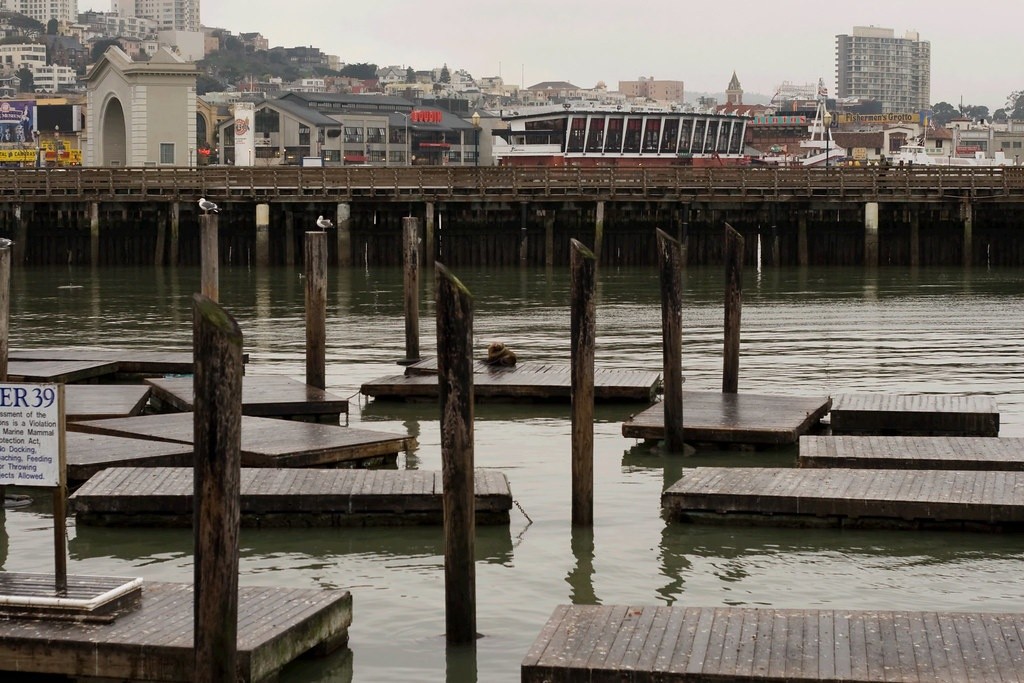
left=471, top=112, right=480, bottom=167
left=824, top=112, right=833, bottom=179
left=189, top=146, right=193, bottom=168
left=35, top=130, right=41, bottom=148
left=393, top=111, right=419, bottom=166
